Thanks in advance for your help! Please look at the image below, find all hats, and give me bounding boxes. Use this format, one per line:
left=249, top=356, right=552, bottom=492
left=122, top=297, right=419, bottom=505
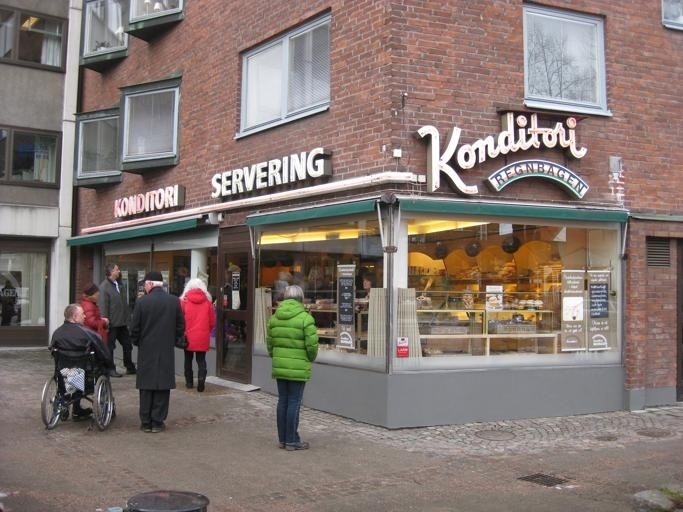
left=82, top=282, right=99, bottom=296
left=138, top=272, right=163, bottom=286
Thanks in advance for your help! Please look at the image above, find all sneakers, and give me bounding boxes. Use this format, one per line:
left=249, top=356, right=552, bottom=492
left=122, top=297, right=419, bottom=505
left=152, top=422, right=166, bottom=432
left=125, top=368, right=137, bottom=374
left=72, top=407, right=92, bottom=422
left=109, top=368, right=123, bottom=378
left=279, top=441, right=310, bottom=451
left=141, top=424, right=151, bottom=433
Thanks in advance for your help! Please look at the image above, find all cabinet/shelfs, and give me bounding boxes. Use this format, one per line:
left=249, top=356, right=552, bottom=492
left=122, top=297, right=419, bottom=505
left=273, top=304, right=368, bottom=355
left=414, top=275, right=562, bottom=329
left=415, top=291, right=558, bottom=355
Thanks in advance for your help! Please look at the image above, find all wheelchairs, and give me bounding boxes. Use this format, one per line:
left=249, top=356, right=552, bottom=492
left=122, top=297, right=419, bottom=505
left=36, top=338, right=116, bottom=430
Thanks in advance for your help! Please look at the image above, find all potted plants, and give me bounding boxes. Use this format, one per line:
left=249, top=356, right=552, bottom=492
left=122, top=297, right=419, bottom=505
left=93, top=41, right=112, bottom=52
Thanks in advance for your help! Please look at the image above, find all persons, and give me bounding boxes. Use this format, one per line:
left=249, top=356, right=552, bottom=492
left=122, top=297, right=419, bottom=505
left=78, top=283, right=110, bottom=344
left=354, top=271, right=377, bottom=314
left=210, top=298, right=240, bottom=367
left=48, top=304, right=113, bottom=418
left=131, top=271, right=185, bottom=432
left=266, top=284, right=319, bottom=452
left=274, top=271, right=289, bottom=294
left=179, top=278, right=215, bottom=392
left=98, top=263, right=137, bottom=378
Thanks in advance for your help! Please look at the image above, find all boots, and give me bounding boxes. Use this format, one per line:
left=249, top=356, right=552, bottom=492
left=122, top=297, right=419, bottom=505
left=197, top=369, right=207, bottom=392
left=185, top=370, right=194, bottom=389
